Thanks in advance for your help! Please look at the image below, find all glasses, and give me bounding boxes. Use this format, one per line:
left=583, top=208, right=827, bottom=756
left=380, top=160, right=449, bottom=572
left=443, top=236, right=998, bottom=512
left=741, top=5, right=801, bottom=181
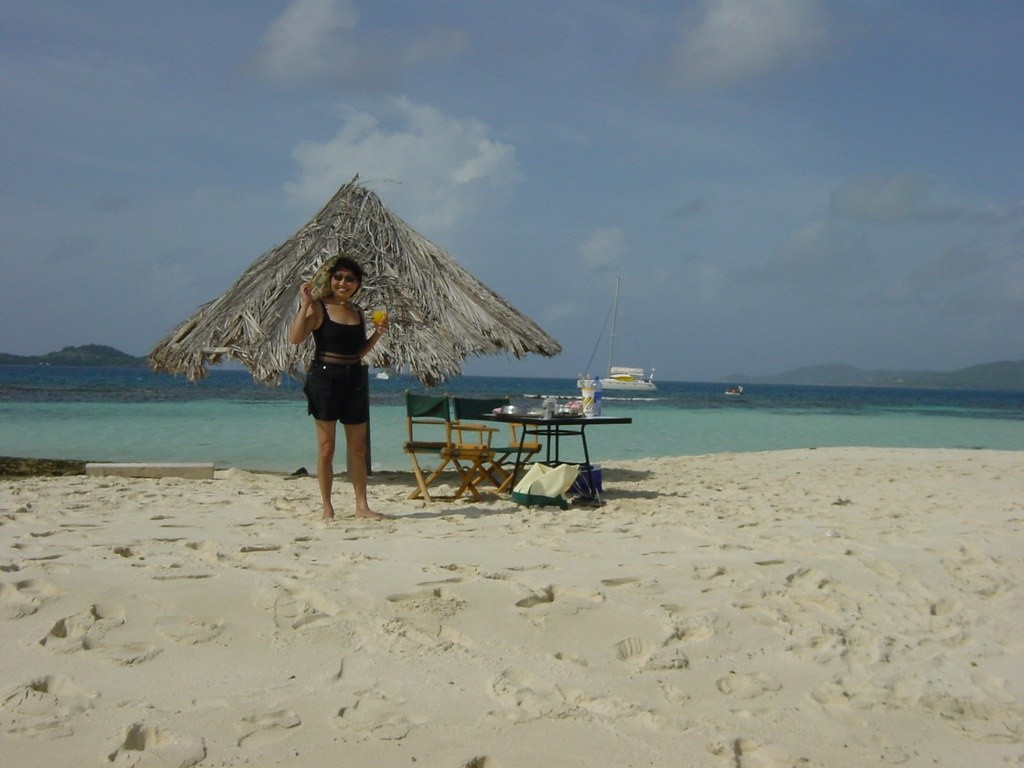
left=332, top=273, right=358, bottom=282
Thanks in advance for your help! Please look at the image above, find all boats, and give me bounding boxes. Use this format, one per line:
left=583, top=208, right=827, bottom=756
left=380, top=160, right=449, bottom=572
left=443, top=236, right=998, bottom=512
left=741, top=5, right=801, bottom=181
left=726, top=386, right=745, bottom=398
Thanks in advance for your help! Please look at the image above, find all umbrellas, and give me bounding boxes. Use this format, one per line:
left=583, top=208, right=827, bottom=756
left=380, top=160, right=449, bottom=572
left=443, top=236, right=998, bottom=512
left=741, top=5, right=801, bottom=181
left=146, top=173, right=563, bottom=392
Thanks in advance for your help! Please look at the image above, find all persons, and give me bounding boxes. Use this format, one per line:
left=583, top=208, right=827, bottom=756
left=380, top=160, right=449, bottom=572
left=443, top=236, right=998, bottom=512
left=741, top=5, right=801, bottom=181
left=290, top=257, right=389, bottom=523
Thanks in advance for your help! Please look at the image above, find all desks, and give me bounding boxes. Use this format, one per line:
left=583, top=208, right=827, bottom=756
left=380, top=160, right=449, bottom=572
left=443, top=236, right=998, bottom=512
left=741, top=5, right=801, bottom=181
left=483, top=414, right=632, bottom=507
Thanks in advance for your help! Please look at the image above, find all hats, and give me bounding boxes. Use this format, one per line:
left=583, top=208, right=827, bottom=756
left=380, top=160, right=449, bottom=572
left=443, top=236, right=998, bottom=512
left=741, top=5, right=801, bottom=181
left=308, top=253, right=364, bottom=302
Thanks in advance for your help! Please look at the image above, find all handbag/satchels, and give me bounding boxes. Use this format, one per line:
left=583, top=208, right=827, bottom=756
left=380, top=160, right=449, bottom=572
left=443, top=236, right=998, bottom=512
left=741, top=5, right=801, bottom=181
left=511, top=462, right=592, bottom=511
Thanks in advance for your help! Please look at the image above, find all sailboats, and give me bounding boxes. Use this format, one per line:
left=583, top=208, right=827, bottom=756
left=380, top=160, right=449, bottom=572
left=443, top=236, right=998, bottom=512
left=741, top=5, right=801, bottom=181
left=576, top=275, right=658, bottom=391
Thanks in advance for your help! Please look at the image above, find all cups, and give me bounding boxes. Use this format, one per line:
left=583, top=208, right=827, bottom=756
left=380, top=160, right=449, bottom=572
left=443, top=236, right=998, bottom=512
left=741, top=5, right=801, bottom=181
left=581, top=388, right=595, bottom=416
left=373, top=308, right=385, bottom=325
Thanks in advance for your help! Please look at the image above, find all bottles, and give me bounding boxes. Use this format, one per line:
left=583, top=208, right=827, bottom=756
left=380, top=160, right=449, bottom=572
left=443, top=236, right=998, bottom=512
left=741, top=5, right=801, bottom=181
left=548, top=396, right=556, bottom=416
left=555, top=404, right=575, bottom=415
left=591, top=376, right=602, bottom=415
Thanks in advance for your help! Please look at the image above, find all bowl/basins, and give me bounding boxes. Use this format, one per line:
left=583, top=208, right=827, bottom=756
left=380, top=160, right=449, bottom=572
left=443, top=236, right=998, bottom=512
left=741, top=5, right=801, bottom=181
left=503, top=406, right=527, bottom=414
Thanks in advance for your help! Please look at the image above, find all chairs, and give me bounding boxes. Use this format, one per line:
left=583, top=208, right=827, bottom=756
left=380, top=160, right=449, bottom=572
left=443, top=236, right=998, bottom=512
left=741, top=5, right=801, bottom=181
left=404, top=390, right=542, bottom=503
